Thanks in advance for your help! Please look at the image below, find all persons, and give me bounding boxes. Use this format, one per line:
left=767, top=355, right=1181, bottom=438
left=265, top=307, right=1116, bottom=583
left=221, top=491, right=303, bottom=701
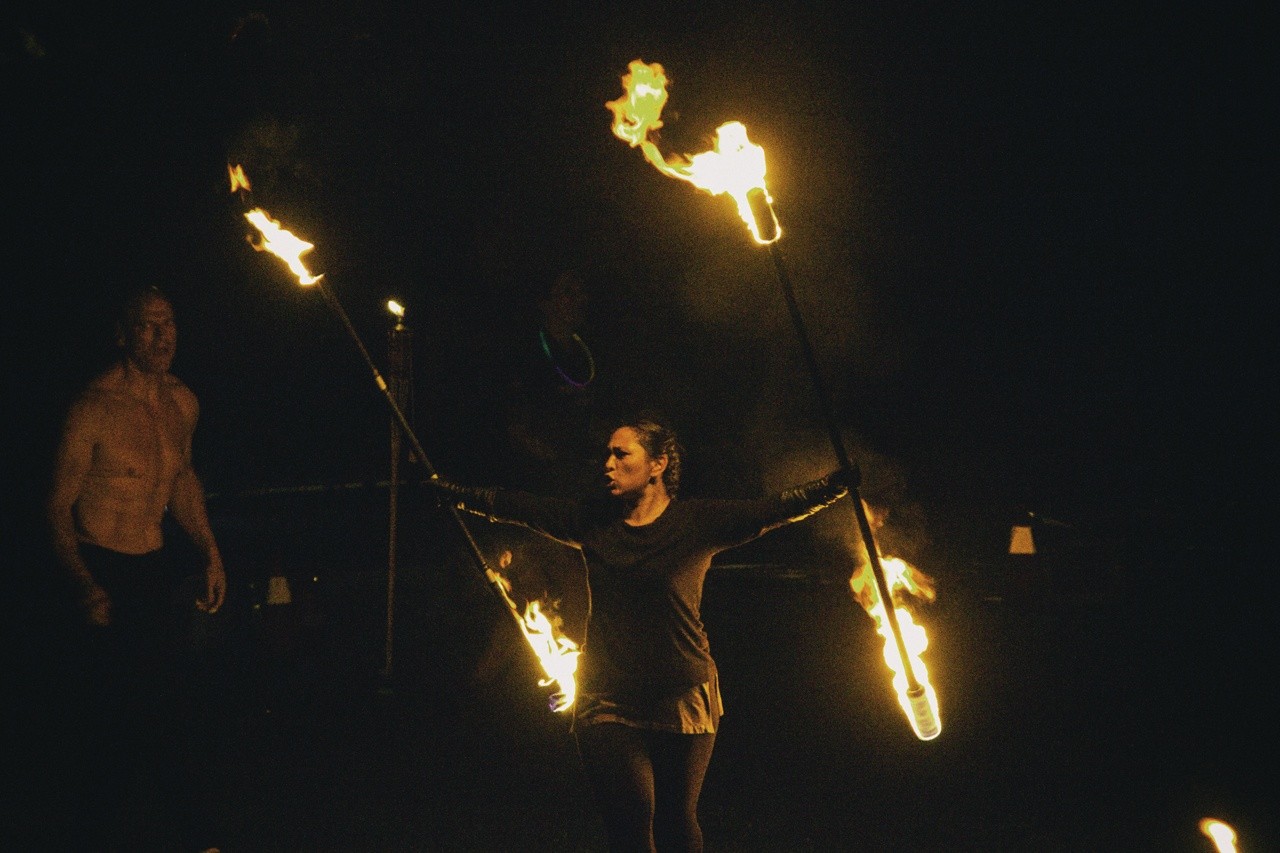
left=476, top=256, right=624, bottom=461
left=49, top=282, right=226, bottom=765
left=420, top=419, right=864, bottom=853
